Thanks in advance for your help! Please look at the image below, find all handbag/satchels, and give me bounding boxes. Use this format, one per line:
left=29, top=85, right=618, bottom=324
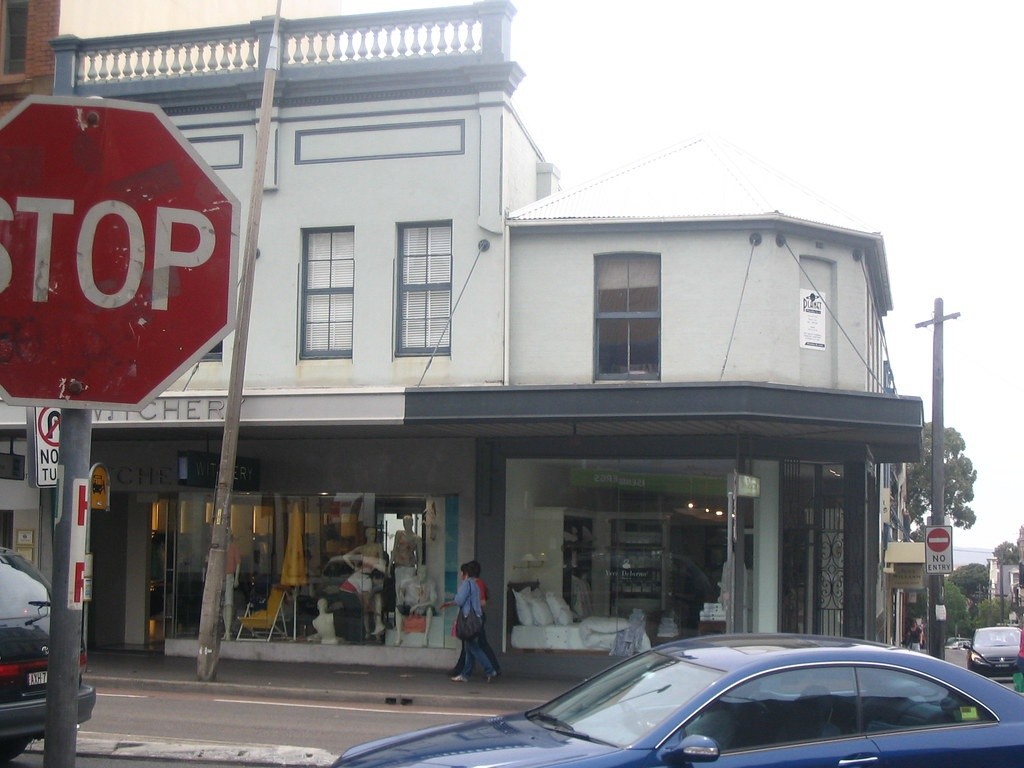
left=1013, top=672, right=1024, bottom=693
left=451, top=618, right=458, bottom=636
left=455, top=605, right=484, bottom=640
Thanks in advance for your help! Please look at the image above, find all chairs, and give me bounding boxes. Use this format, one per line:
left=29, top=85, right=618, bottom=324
left=235, top=584, right=290, bottom=643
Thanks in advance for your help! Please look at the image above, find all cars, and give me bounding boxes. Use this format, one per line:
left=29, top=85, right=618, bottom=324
left=962, top=625, right=1023, bottom=677
left=318, top=558, right=393, bottom=616
left=944, top=637, right=971, bottom=651
left=325, top=632, right=1023, bottom=768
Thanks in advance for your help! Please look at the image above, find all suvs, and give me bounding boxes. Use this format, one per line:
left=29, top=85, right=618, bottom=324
left=0, top=547, right=98, bottom=765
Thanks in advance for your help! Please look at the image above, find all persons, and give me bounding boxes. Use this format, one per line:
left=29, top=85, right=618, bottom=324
left=1013, top=624, right=1024, bottom=693
left=203, top=527, right=241, bottom=641
left=311, top=598, right=340, bottom=645
left=387, top=515, right=423, bottom=646
left=342, top=528, right=387, bottom=639
left=440, top=561, right=502, bottom=684
left=908, top=621, right=922, bottom=652
left=681, top=696, right=737, bottom=751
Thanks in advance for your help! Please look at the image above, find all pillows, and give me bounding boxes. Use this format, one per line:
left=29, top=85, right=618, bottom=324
left=527, top=589, right=554, bottom=627
left=547, top=591, right=570, bottom=625
left=511, top=586, right=539, bottom=626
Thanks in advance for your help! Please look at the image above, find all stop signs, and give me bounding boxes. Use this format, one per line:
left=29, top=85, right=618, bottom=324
left=928, top=527, right=950, bottom=553
left=0, top=92, right=241, bottom=411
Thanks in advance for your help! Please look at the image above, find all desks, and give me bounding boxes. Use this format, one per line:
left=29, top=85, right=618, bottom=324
left=696, top=619, right=727, bottom=637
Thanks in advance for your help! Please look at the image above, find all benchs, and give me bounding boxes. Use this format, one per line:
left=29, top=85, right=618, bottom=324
left=869, top=679, right=959, bottom=729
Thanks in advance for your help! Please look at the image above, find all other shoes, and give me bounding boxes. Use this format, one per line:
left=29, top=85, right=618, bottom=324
left=451, top=674, right=468, bottom=683
left=483, top=670, right=497, bottom=682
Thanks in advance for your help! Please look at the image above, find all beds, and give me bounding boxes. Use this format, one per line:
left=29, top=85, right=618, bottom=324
left=508, top=582, right=654, bottom=680
